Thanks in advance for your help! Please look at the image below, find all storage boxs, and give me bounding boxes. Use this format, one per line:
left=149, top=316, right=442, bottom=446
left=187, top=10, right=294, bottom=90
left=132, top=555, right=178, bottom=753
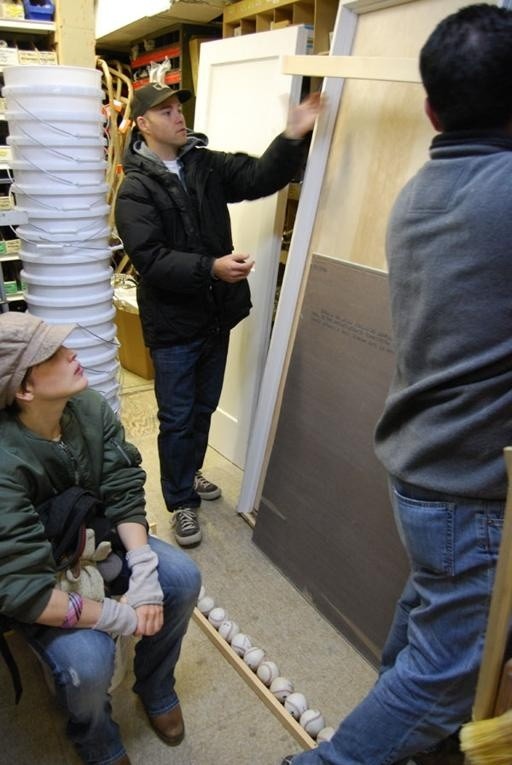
left=113, top=286, right=157, bottom=380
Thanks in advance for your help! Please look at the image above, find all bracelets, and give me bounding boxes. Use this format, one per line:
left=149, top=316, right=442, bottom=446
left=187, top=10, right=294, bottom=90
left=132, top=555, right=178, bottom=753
left=61, top=591, right=85, bottom=630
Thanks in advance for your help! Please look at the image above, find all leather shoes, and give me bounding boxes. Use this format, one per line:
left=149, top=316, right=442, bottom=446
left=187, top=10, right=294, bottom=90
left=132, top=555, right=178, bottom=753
left=144, top=689, right=185, bottom=746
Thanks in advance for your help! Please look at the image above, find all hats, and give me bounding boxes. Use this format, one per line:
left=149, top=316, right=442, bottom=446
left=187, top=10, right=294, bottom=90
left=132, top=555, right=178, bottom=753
left=130, top=81, right=192, bottom=116
left=0, top=310, right=79, bottom=406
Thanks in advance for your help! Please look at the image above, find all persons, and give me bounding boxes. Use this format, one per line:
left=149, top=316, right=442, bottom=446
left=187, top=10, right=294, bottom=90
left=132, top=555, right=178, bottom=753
left=283, top=2, right=510, bottom=765
left=0, top=308, right=203, bottom=765
left=111, top=77, right=329, bottom=546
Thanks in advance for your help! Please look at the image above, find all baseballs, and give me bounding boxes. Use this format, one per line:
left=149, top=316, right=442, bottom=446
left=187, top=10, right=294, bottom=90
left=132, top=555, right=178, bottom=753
left=197, top=583, right=267, bottom=670
left=255, top=662, right=336, bottom=745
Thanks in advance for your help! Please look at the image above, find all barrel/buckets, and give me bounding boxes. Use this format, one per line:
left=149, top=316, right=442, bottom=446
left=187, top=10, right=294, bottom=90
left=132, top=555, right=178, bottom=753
left=2, top=65, right=125, bottom=423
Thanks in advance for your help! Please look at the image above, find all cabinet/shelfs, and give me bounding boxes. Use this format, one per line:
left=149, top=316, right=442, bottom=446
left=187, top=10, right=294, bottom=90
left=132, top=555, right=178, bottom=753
left=222, top=0, right=339, bottom=266
left=129, top=23, right=222, bottom=130
left=0, top=19, right=60, bottom=312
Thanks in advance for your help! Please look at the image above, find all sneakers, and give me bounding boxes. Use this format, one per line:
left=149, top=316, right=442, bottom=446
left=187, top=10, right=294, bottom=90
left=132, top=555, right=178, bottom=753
left=193, top=466, right=221, bottom=500
left=171, top=504, right=203, bottom=546
